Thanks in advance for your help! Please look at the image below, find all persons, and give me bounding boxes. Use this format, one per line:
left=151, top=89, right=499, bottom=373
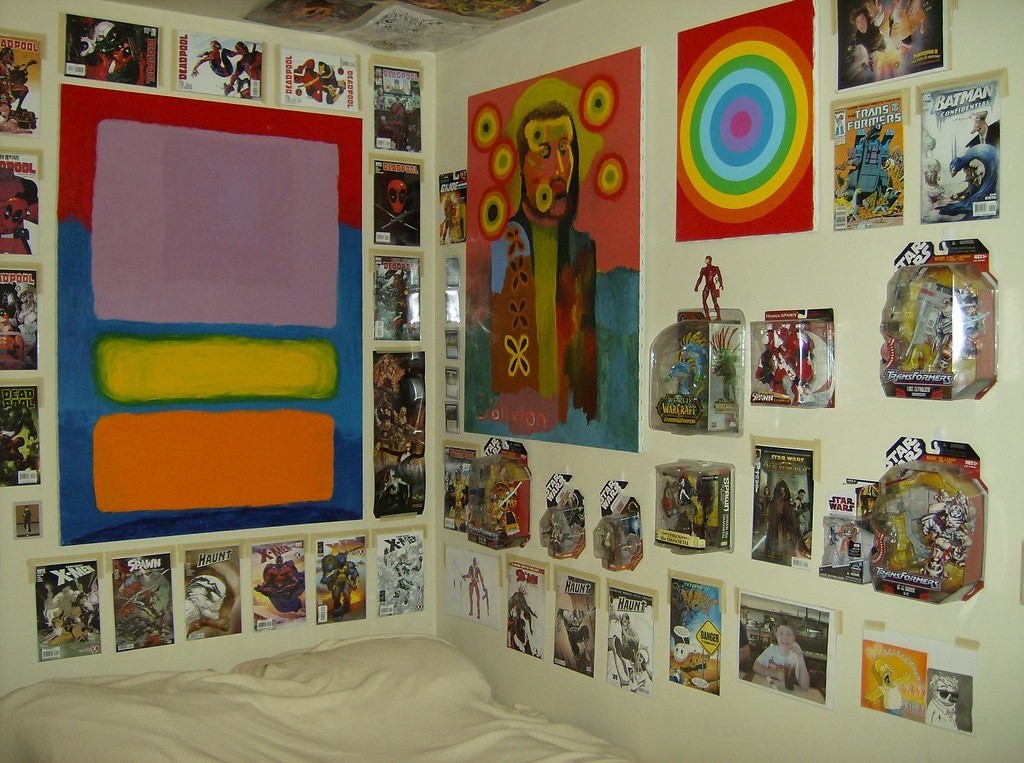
left=738, top=618, right=810, bottom=691
left=756, top=479, right=809, bottom=562
left=694, top=256, right=724, bottom=321
left=860, top=486, right=877, bottom=525
left=846, top=0, right=926, bottom=71
